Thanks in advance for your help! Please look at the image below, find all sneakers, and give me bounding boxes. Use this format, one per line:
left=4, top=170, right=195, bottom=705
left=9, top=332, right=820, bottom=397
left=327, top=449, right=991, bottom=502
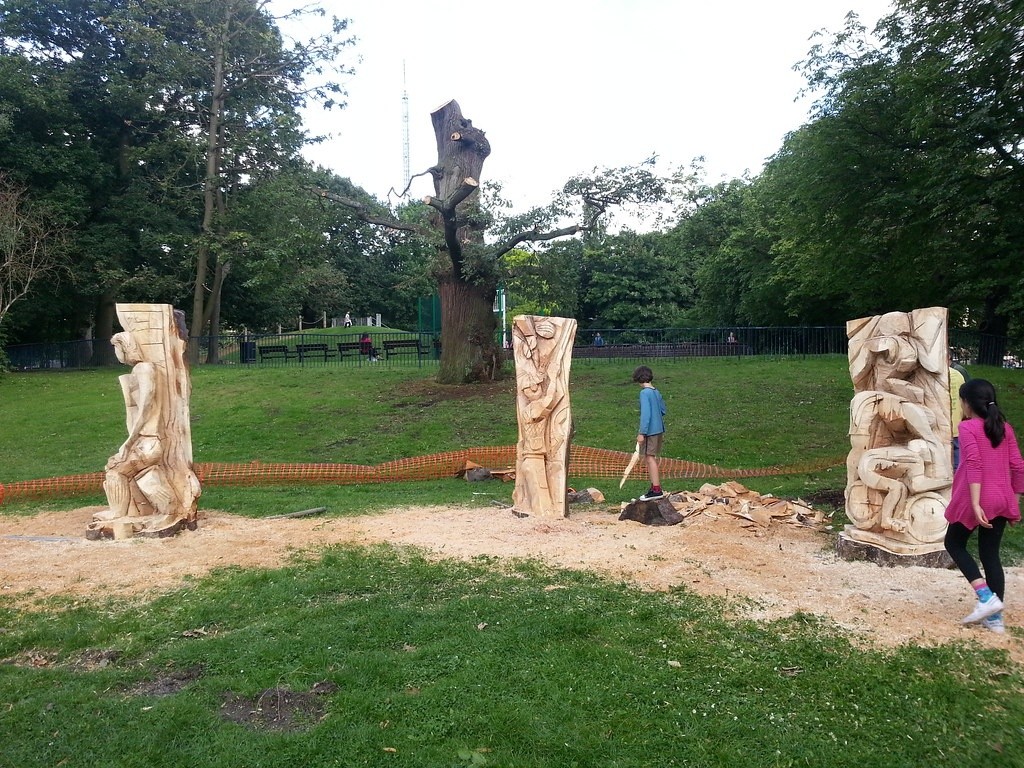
left=963, top=592, right=1005, bottom=623
left=639, top=489, right=663, bottom=501
left=983, top=618, right=1004, bottom=633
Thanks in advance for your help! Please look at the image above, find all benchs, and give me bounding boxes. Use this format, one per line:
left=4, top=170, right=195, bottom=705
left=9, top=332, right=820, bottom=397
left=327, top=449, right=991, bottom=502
left=296, top=343, right=337, bottom=362
left=257, top=345, right=298, bottom=363
left=337, top=342, right=382, bottom=361
left=382, top=339, right=431, bottom=360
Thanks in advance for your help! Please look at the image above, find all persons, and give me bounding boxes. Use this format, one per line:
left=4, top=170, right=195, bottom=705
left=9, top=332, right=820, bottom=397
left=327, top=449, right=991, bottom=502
left=633, top=365, right=667, bottom=502
left=944, top=378, right=1024, bottom=633
left=359, top=332, right=385, bottom=361
left=949, top=367, right=966, bottom=475
left=949, top=346, right=971, bottom=383
left=593, top=332, right=603, bottom=346
left=344, top=311, right=353, bottom=326
left=727, top=332, right=738, bottom=343
